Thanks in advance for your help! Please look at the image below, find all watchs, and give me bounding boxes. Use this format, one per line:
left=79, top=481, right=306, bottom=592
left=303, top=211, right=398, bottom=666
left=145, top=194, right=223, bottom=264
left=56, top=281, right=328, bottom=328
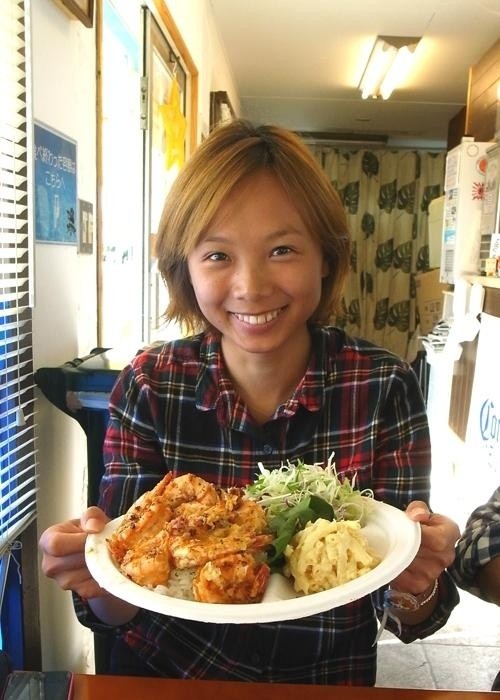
left=381, top=576, right=440, bottom=613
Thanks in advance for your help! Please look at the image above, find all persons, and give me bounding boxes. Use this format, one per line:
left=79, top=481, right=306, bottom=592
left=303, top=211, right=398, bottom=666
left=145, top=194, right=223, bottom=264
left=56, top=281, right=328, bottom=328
left=37, top=117, right=462, bottom=688
left=448, top=482, right=500, bottom=693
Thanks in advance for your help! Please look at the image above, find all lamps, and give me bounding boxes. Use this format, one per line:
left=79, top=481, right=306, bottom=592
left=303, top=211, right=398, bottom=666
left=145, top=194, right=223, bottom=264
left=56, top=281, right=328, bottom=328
left=357, top=35, right=421, bottom=101
left=293, top=132, right=389, bottom=151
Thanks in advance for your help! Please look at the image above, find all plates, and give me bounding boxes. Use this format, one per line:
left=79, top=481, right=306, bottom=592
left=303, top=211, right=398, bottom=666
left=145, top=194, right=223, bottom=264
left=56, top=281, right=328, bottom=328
left=82, top=473, right=423, bottom=625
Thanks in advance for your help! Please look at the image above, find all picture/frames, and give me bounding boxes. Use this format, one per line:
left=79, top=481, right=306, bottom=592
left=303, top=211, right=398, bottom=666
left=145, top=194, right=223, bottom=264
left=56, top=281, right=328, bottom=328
left=50, top=0, right=94, bottom=28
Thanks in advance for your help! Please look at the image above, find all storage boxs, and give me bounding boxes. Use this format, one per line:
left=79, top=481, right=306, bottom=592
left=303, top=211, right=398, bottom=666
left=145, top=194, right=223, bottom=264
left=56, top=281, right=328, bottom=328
left=415, top=267, right=453, bottom=336
